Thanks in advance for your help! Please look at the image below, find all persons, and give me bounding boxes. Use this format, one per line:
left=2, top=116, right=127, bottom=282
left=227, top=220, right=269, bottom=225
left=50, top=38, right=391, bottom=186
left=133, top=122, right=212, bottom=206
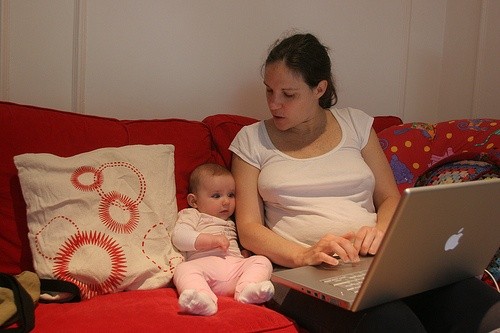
left=228, top=33, right=500, bottom=333
left=171, top=163, right=275, bottom=316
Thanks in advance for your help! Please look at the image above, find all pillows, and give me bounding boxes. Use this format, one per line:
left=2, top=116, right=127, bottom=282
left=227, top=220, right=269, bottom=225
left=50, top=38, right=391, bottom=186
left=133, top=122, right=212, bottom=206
left=14, top=144, right=187, bottom=299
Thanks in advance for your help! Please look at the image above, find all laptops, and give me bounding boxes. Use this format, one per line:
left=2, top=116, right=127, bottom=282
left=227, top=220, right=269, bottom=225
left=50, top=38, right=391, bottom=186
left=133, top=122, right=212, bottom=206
left=271, top=178, right=500, bottom=312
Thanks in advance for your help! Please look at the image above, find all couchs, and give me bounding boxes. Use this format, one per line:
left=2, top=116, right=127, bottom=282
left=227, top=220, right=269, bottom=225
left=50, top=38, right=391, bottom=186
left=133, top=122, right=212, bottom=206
left=1, top=101, right=500, bottom=333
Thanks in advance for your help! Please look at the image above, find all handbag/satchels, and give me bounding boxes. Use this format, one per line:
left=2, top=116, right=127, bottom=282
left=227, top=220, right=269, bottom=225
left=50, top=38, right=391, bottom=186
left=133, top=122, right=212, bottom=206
left=0, top=270, right=82, bottom=332
